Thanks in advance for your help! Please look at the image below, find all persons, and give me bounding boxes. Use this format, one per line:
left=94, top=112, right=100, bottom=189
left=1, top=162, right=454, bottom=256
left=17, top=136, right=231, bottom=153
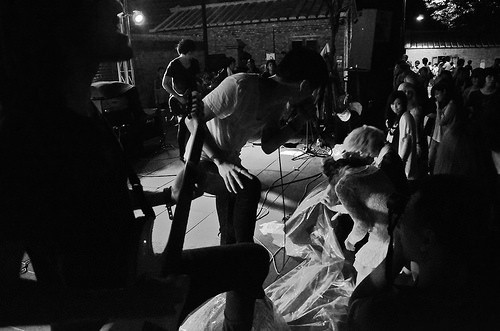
left=0, top=0, right=270, bottom=331
left=162, top=39, right=200, bottom=164
left=247, top=58, right=260, bottom=73
left=225, top=55, right=233, bottom=77
left=324, top=53, right=500, bottom=331
left=154, top=67, right=168, bottom=108
left=177, top=48, right=329, bottom=245
left=262, top=58, right=277, bottom=77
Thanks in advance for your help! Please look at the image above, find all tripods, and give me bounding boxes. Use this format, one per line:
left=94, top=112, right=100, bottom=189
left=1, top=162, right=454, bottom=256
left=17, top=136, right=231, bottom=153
left=293, top=119, right=327, bottom=159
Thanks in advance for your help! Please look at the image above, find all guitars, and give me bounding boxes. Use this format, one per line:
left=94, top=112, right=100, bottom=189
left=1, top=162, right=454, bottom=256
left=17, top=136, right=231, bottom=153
left=98, top=91, right=208, bottom=331
left=168, top=67, right=225, bottom=117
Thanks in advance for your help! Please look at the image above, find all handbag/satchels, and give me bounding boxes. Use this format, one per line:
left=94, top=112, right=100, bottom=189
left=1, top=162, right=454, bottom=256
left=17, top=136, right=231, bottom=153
left=284, top=177, right=338, bottom=246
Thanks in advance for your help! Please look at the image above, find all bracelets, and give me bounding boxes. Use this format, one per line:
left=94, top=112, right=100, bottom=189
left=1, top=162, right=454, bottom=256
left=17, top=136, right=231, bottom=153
left=209, top=149, right=223, bottom=163
